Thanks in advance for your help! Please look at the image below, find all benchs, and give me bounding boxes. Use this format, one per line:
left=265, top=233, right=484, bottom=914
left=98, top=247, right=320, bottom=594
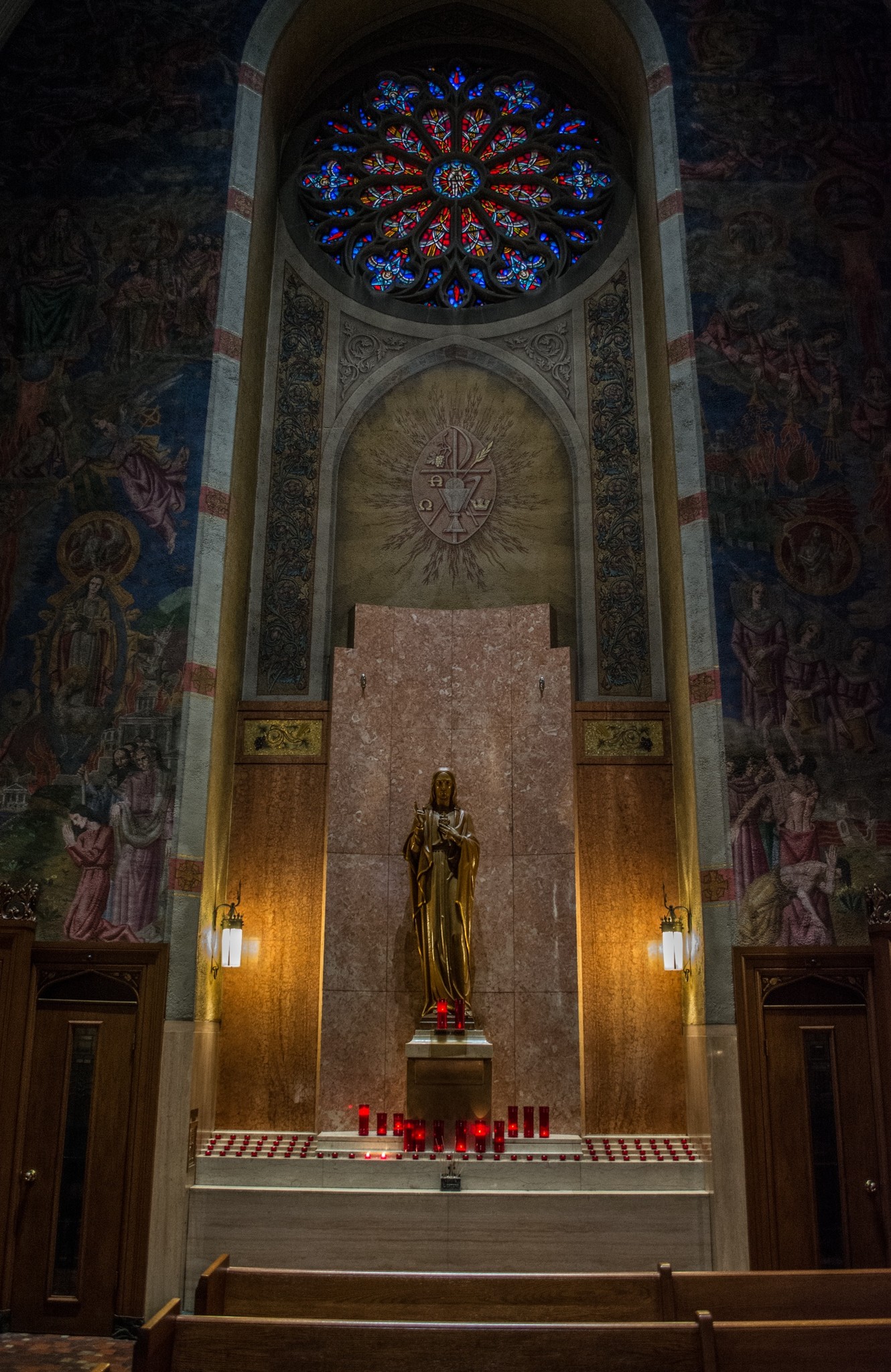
left=134, top=1253, right=891, bottom=1372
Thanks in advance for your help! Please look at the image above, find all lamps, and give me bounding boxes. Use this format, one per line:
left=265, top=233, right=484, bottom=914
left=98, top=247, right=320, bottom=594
left=208, top=879, right=244, bottom=979
left=659, top=882, right=690, bottom=981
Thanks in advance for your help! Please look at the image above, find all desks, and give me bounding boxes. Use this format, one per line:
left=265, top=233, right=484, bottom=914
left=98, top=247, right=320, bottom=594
left=183, top=1187, right=716, bottom=1312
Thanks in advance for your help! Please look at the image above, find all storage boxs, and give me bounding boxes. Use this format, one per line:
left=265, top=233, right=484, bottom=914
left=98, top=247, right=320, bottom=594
left=440, top=1173, right=462, bottom=1192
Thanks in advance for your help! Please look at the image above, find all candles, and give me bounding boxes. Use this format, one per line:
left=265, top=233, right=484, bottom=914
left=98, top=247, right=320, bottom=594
left=559, top=1137, right=695, bottom=1161
left=204, top=1133, right=313, bottom=1158
left=455, top=999, right=465, bottom=1029
left=437, top=999, right=447, bottom=1029
left=317, top=1104, right=549, bottom=1161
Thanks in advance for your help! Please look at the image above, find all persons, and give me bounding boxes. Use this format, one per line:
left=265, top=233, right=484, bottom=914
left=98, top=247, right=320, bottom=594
left=402, top=767, right=480, bottom=1021
